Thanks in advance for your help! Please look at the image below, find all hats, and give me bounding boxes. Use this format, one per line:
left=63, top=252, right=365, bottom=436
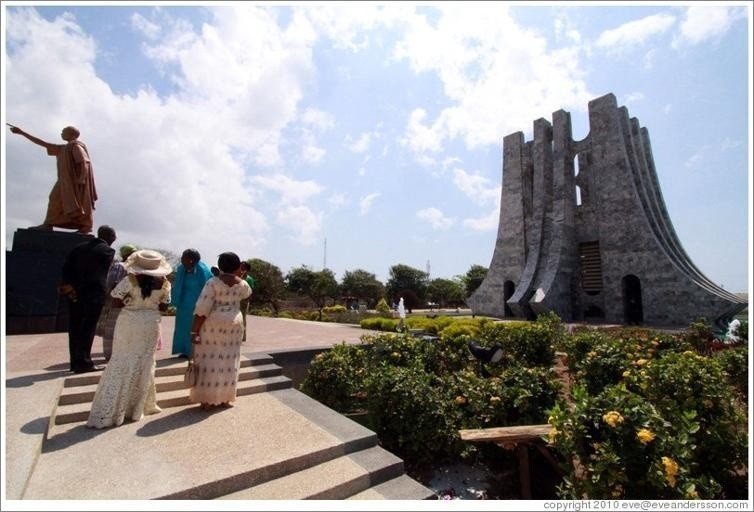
left=118, top=243, right=174, bottom=278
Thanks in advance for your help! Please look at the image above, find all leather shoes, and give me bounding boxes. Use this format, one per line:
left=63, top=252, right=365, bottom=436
left=75, top=363, right=105, bottom=374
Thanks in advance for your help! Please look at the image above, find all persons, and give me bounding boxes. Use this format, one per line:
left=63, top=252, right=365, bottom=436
left=189, top=252, right=253, bottom=412
left=170, top=248, right=215, bottom=358
left=85, top=248, right=172, bottom=430
left=53, top=224, right=114, bottom=374
left=94, top=242, right=137, bottom=362
left=236, top=260, right=257, bottom=342
left=6, top=123, right=97, bottom=234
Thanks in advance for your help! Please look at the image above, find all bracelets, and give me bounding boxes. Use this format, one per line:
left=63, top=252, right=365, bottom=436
left=190, top=331, right=198, bottom=335
